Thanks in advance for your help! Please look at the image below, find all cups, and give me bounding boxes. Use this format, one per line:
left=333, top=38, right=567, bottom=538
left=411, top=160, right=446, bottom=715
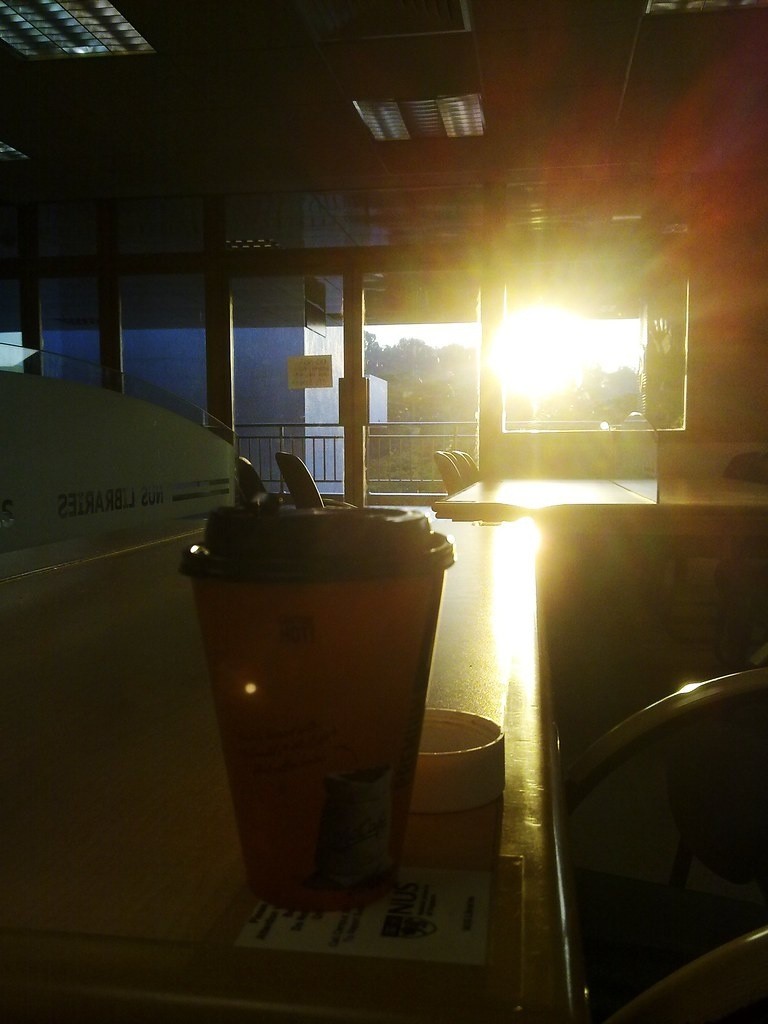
left=180, top=501, right=455, bottom=910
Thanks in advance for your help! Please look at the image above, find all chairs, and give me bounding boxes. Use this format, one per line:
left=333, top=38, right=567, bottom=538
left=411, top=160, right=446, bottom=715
left=434, top=451, right=480, bottom=496
left=236, top=451, right=356, bottom=508
left=0, top=477, right=768, bottom=1024
left=574, top=451, right=768, bottom=1024
